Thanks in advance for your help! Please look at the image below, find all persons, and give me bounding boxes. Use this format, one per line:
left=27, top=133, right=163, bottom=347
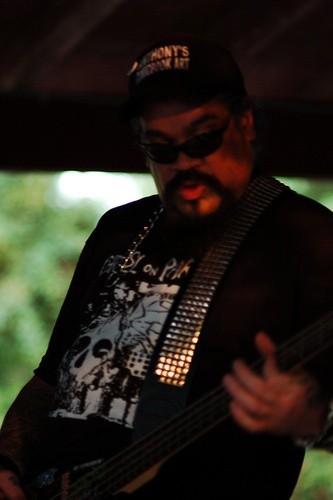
left=0, top=36, right=333, bottom=500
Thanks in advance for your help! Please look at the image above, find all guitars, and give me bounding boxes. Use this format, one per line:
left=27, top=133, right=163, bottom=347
left=27, top=308, right=333, bottom=500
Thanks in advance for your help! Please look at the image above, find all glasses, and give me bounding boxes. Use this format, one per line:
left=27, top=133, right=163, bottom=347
left=136, top=113, right=233, bottom=165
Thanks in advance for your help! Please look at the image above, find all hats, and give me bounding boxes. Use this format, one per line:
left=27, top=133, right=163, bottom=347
left=127, top=27, right=245, bottom=126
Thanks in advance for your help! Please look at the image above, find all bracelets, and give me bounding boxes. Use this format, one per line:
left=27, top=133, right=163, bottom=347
left=295, top=402, right=333, bottom=447
